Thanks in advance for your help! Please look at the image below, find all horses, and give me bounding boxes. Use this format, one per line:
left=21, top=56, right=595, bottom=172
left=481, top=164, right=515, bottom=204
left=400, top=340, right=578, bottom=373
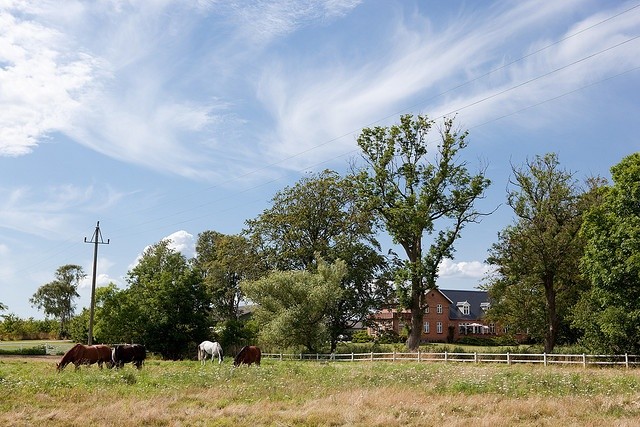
left=198, top=340, right=224, bottom=367
left=233, top=345, right=261, bottom=367
left=55, top=343, right=112, bottom=373
left=112, top=343, right=146, bottom=369
